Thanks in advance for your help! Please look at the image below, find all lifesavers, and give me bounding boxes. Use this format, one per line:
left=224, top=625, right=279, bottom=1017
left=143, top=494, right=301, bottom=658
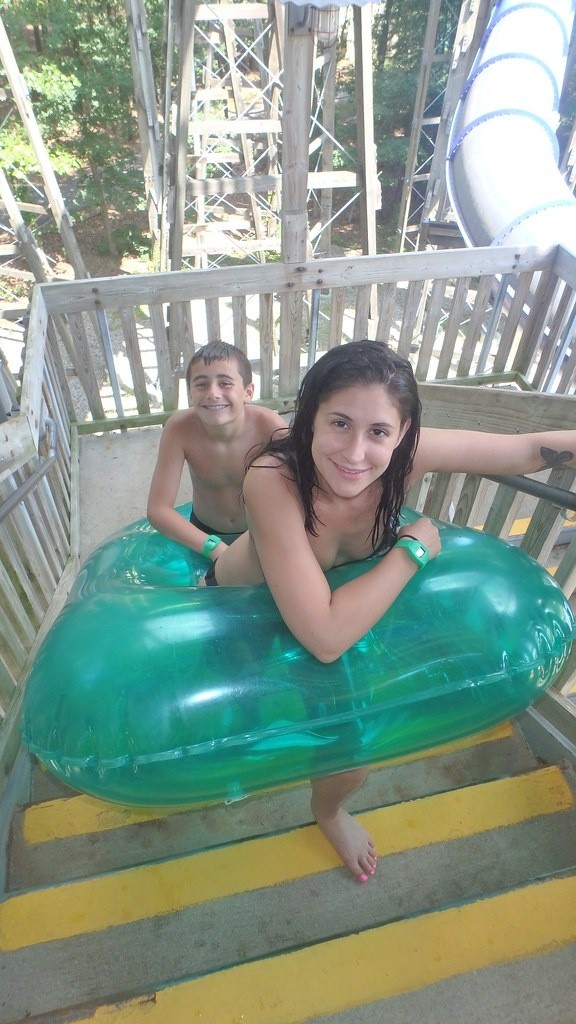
left=15, top=496, right=576, bottom=812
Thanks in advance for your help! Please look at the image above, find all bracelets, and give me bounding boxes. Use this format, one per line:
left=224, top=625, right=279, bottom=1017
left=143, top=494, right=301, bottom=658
left=395, top=534, right=421, bottom=543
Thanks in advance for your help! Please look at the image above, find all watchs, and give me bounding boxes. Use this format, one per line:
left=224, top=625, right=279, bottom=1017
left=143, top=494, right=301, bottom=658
left=201, top=534, right=223, bottom=560
left=389, top=535, right=430, bottom=574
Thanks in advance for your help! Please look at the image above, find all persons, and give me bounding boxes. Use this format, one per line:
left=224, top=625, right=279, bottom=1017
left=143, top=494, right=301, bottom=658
left=144, top=340, right=289, bottom=563
left=200, top=340, right=576, bottom=884
